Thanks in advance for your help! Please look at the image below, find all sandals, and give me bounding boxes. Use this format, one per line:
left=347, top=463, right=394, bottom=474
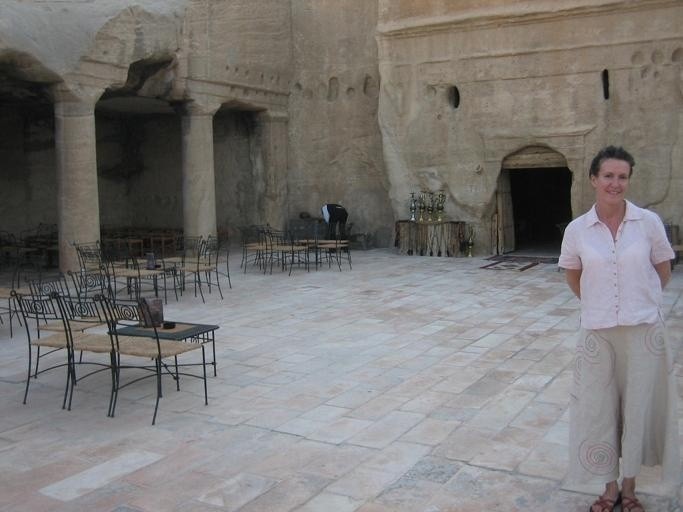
left=590, top=493, right=620, bottom=508
left=619, top=494, right=643, bottom=508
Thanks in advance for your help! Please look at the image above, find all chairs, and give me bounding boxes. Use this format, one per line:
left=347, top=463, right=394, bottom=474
left=241, top=221, right=353, bottom=277
left=103, top=241, right=167, bottom=299
left=101, top=296, right=211, bottom=424
left=27, top=273, right=112, bottom=382
left=73, top=239, right=130, bottom=300
left=11, top=291, right=138, bottom=410
left=173, top=239, right=224, bottom=304
left=51, top=292, right=163, bottom=418
left=187, top=234, right=232, bottom=294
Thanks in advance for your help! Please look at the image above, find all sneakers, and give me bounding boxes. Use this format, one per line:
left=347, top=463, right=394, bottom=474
left=327, top=250, right=335, bottom=253
left=344, top=250, right=349, bottom=252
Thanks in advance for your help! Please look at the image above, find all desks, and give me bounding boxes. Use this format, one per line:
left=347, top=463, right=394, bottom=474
left=108, top=316, right=220, bottom=391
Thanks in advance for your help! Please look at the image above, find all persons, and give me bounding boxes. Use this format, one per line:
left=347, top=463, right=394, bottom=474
left=557, top=145, right=675, bottom=512
left=321, top=203, right=350, bottom=253
